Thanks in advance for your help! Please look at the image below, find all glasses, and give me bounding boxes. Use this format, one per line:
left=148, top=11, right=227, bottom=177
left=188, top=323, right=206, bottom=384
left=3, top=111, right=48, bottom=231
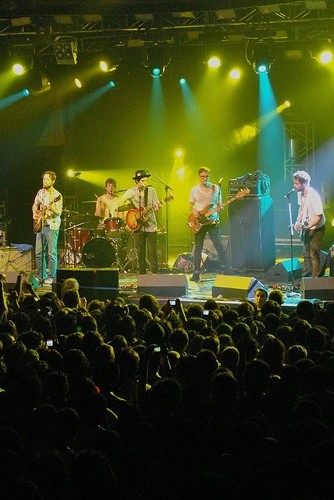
left=200, top=176, right=209, bottom=179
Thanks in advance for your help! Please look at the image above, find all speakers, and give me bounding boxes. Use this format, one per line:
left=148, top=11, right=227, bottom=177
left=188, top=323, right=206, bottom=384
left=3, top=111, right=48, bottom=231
left=136, top=274, right=189, bottom=296
left=300, top=276, right=334, bottom=301
left=192, top=238, right=230, bottom=265
left=212, top=275, right=266, bottom=300
left=3, top=271, right=31, bottom=294
left=228, top=195, right=276, bottom=271
left=265, top=258, right=303, bottom=282
left=52, top=268, right=120, bottom=306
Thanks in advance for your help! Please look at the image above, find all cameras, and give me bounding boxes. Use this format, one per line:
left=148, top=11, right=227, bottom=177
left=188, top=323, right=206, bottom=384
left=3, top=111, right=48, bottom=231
left=47, top=341, right=53, bottom=347
left=169, top=300, right=176, bottom=305
left=202, top=310, right=209, bottom=315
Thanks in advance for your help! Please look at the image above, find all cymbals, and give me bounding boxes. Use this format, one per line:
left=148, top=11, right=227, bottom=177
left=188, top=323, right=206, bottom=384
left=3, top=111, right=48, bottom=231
left=62, top=210, right=79, bottom=215
left=115, top=204, right=133, bottom=212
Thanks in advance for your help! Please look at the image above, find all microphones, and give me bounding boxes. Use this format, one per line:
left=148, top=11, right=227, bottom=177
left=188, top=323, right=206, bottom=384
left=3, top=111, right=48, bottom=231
left=43, top=184, right=47, bottom=188
left=284, top=188, right=295, bottom=198
left=138, top=184, right=145, bottom=189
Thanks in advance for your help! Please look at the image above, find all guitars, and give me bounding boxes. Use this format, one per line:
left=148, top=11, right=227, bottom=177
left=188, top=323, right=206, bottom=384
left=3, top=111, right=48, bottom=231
left=188, top=187, right=250, bottom=234
left=32, top=194, right=61, bottom=234
left=297, top=220, right=309, bottom=242
left=126, top=195, right=174, bottom=231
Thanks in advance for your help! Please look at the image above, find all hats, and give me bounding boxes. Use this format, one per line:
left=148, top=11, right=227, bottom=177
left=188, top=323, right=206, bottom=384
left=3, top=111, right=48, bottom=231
left=132, top=170, right=151, bottom=179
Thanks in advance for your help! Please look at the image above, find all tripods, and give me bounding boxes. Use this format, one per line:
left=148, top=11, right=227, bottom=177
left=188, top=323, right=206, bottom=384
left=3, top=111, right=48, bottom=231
left=58, top=212, right=89, bottom=269
left=113, top=233, right=151, bottom=273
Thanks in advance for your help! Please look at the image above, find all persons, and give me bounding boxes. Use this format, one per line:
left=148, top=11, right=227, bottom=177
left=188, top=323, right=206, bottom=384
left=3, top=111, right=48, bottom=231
left=0, top=272, right=334, bottom=500
left=293, top=170, right=326, bottom=286
left=94, top=177, right=128, bottom=252
left=121, top=170, right=162, bottom=275
left=190, top=167, right=233, bottom=280
left=32, top=171, right=63, bottom=285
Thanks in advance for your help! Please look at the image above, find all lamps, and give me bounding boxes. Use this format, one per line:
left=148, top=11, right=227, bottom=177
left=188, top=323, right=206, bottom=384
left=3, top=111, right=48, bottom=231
left=52, top=36, right=78, bottom=65
left=147, top=54, right=170, bottom=77
left=253, top=54, right=271, bottom=74
left=99, top=48, right=126, bottom=72
left=75, top=72, right=93, bottom=88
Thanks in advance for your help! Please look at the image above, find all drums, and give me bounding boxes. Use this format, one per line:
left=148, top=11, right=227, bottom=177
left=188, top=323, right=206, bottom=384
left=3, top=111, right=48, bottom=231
left=60, top=220, right=73, bottom=238
left=69, top=228, right=89, bottom=251
left=81, top=238, right=116, bottom=268
left=104, top=217, right=125, bottom=238
left=90, top=229, right=105, bottom=237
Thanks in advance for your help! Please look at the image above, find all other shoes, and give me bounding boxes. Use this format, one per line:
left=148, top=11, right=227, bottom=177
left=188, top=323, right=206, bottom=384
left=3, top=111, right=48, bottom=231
left=43, top=277, right=53, bottom=286
left=190, top=273, right=199, bottom=281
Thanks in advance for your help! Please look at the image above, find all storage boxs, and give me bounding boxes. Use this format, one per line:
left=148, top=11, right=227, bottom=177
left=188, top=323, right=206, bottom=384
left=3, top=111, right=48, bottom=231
left=229, top=177, right=271, bottom=196
